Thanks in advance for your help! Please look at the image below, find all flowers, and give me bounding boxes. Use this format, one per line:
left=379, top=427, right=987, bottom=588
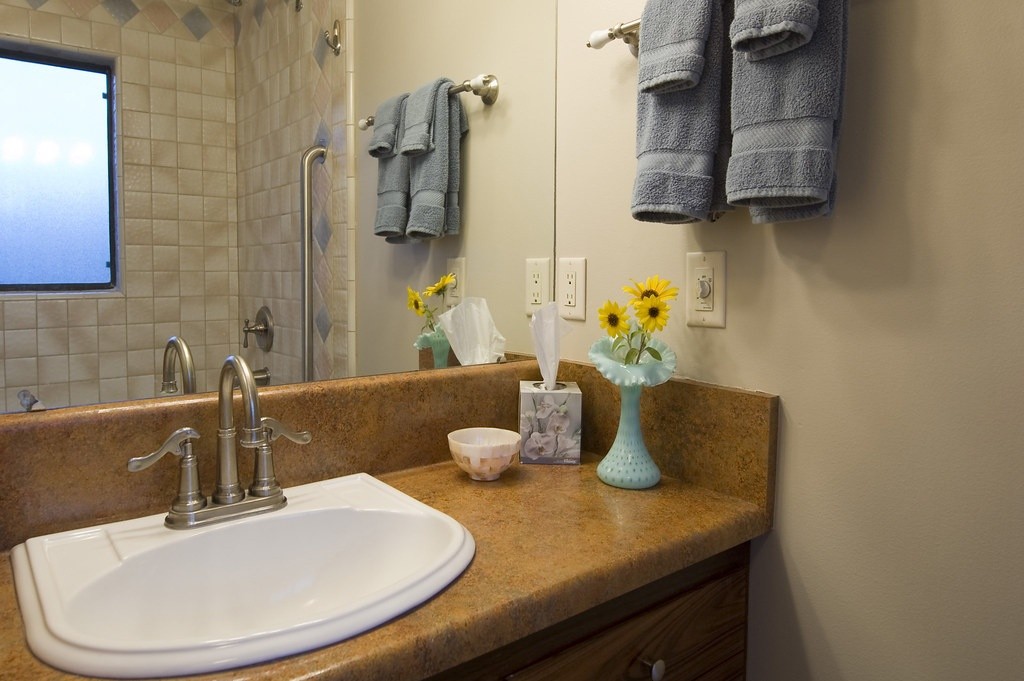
left=406, top=273, right=456, bottom=334
left=596, top=275, right=679, bottom=363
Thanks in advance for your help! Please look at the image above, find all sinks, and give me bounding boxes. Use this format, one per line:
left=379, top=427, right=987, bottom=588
left=9, top=472, right=476, bottom=678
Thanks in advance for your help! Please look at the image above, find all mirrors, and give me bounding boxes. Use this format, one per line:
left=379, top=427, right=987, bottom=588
left=0, top=0, right=558, bottom=416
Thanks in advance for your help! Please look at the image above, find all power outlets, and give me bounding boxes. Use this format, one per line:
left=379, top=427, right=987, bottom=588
left=526, top=257, right=550, bottom=317
left=557, top=257, right=586, bottom=321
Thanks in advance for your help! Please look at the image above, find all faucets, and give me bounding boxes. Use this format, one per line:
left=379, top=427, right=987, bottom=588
left=157, top=335, right=199, bottom=394
left=219, top=356, right=265, bottom=500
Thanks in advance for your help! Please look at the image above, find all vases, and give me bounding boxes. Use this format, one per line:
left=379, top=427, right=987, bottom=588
left=589, top=334, right=677, bottom=490
left=413, top=322, right=451, bottom=370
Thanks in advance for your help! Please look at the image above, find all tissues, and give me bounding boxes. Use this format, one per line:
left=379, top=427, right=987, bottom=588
left=519, top=302, right=582, bottom=465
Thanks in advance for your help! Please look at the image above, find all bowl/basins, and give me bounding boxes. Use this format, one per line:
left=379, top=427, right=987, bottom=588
left=446, top=426, right=522, bottom=482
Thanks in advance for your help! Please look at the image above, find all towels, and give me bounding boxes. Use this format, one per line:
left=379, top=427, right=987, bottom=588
left=367, top=76, right=470, bottom=244
left=725, top=0, right=852, bottom=224
left=630, top=0, right=726, bottom=225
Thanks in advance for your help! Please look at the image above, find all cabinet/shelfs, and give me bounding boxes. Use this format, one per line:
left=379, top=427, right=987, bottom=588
left=423, top=544, right=751, bottom=681
